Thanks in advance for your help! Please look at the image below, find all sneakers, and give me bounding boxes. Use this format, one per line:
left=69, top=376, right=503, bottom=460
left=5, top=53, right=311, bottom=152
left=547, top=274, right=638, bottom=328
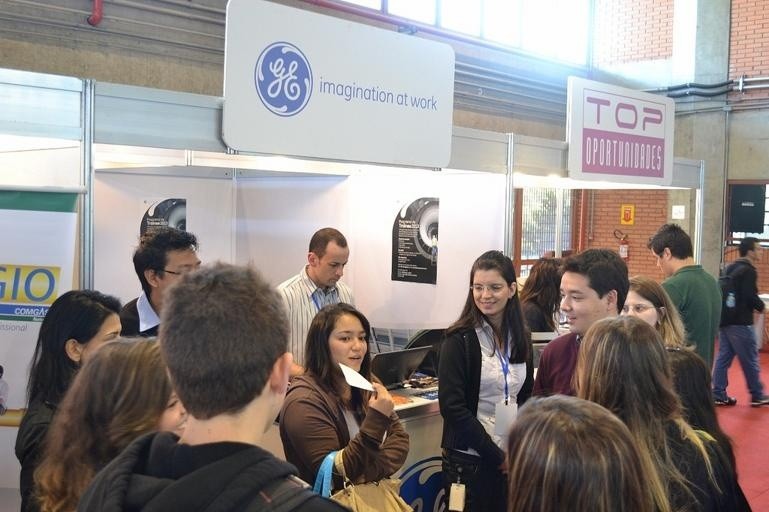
left=751, top=396, right=769, bottom=406
left=714, top=397, right=736, bottom=406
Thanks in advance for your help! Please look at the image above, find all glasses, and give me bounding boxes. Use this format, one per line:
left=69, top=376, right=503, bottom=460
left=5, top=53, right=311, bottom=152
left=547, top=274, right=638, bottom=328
left=624, top=305, right=655, bottom=312
left=470, top=283, right=504, bottom=291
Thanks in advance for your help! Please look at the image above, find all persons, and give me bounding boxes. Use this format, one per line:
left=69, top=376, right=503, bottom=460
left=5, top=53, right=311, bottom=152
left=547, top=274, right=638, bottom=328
left=121, top=228, right=201, bottom=337
left=438, top=248, right=534, bottom=512
left=508, top=224, right=753, bottom=512
left=15, top=290, right=123, bottom=510
left=713, top=236, right=769, bottom=407
left=32, top=334, right=189, bottom=511
left=279, top=301, right=409, bottom=496
left=78, top=262, right=355, bottom=511
left=274, top=227, right=357, bottom=393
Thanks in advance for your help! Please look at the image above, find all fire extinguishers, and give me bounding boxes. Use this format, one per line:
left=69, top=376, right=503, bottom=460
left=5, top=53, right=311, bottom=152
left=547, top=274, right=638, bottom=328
left=614, top=229, right=629, bottom=262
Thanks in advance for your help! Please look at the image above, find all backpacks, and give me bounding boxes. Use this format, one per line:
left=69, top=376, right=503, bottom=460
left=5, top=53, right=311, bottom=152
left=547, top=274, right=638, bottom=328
left=719, top=263, right=748, bottom=326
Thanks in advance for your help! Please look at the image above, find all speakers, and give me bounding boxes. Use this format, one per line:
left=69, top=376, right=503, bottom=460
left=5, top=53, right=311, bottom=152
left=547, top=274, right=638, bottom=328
left=728, top=183, right=767, bottom=233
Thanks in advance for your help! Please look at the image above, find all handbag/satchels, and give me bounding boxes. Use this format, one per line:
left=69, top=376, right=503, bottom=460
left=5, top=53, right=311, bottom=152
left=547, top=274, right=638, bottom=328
left=329, top=478, right=413, bottom=512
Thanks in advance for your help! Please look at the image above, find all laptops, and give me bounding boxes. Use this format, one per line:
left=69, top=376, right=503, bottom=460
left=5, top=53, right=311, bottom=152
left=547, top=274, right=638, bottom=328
left=366, top=344, right=431, bottom=393
